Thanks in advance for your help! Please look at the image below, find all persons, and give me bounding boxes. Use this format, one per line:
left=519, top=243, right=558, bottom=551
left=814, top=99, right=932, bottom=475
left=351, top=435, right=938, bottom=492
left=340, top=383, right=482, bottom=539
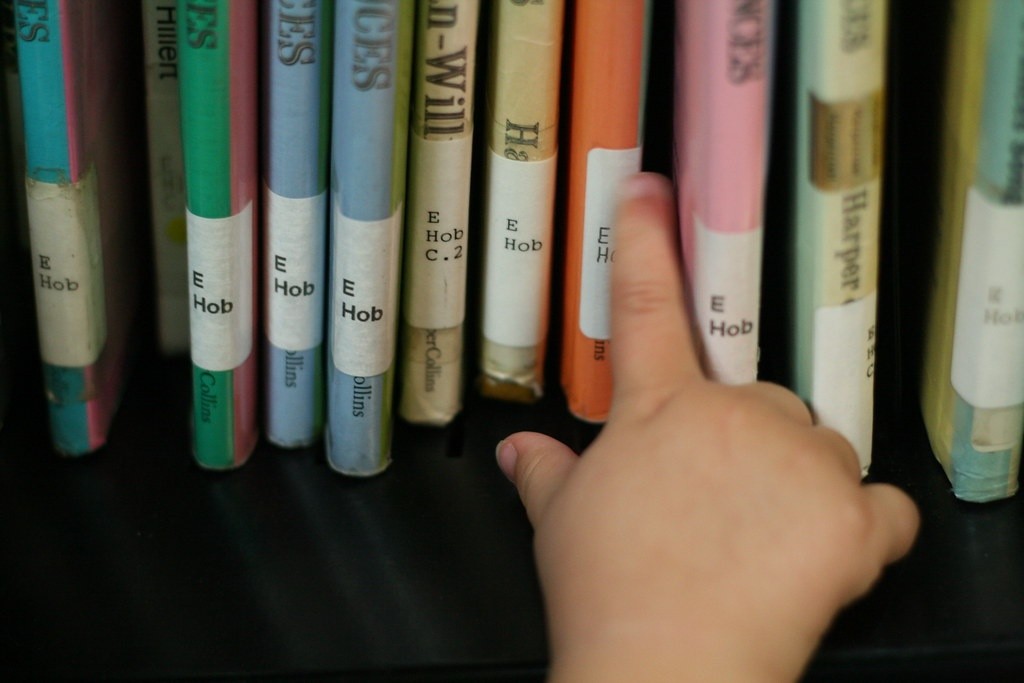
left=494, top=174, right=919, bottom=683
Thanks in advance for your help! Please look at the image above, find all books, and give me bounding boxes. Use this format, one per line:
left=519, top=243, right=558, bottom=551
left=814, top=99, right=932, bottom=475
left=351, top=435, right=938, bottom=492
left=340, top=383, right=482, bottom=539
left=16, top=0, right=1024, bottom=504
